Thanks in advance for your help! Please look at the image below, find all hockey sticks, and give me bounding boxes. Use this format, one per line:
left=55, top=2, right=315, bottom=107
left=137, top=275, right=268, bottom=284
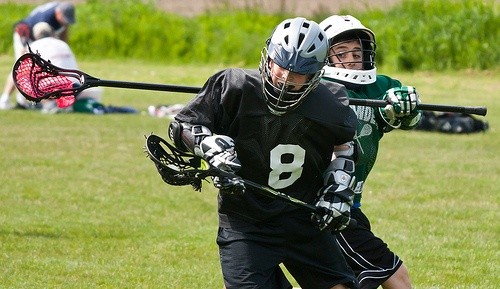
left=13, top=42, right=487, bottom=117
left=142, top=131, right=320, bottom=215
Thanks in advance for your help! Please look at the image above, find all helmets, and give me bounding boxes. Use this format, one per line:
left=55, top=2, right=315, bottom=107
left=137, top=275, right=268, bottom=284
left=318, top=14, right=378, bottom=83
left=267, top=17, right=329, bottom=101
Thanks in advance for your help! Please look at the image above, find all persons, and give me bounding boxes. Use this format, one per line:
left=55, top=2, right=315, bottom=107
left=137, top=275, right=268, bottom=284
left=168, top=16, right=359, bottom=289
left=314, top=12, right=422, bottom=289
left=0, top=1, right=74, bottom=109
left=18, top=20, right=100, bottom=111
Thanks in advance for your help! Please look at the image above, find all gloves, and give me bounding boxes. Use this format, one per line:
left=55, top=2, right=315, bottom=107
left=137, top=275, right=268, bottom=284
left=199, top=135, right=245, bottom=195
left=311, top=173, right=359, bottom=231
left=382, top=86, right=421, bottom=129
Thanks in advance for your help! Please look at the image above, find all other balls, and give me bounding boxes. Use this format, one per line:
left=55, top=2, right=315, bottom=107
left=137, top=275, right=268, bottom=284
left=166, top=163, right=178, bottom=171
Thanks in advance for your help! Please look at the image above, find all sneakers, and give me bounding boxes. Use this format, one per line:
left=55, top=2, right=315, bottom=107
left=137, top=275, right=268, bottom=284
left=41, top=101, right=61, bottom=113
left=1, top=99, right=16, bottom=109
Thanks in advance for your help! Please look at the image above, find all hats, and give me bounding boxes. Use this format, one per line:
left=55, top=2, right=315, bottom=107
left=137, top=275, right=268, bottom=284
left=60, top=4, right=76, bottom=24
